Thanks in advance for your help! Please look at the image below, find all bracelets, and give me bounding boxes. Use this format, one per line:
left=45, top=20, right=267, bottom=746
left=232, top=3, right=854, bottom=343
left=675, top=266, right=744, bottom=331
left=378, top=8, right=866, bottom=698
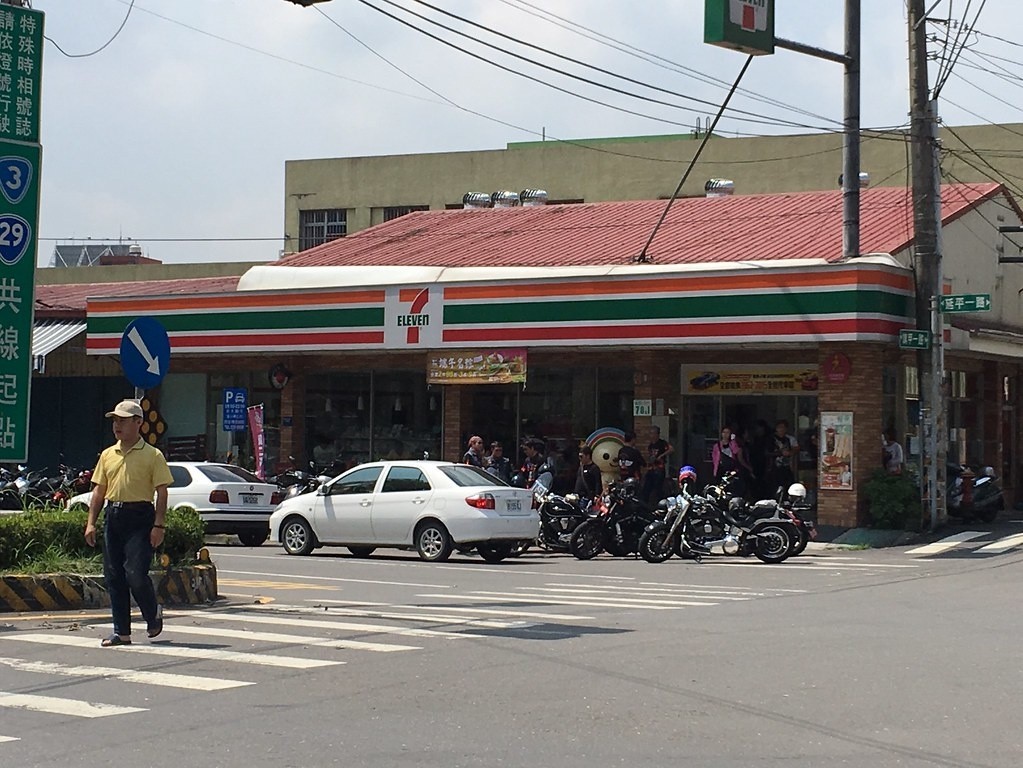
left=153, top=525, right=166, bottom=530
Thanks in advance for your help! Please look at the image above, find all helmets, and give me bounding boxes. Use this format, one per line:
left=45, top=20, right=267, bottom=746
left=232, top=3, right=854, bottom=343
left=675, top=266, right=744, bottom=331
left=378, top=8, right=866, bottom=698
left=788, top=483, right=806, bottom=498
left=624, top=478, right=639, bottom=495
left=728, top=497, right=745, bottom=515
left=512, top=474, right=526, bottom=487
left=679, top=466, right=697, bottom=483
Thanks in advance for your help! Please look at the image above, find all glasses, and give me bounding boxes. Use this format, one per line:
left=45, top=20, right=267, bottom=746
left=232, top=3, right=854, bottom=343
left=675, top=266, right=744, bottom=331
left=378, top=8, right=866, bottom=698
left=493, top=449, right=502, bottom=452
left=579, top=454, right=586, bottom=459
left=476, top=441, right=483, bottom=445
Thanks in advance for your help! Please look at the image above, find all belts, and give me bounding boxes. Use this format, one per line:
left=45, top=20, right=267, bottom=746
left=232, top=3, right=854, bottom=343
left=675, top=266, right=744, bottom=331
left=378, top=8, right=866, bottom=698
left=108, top=501, right=152, bottom=508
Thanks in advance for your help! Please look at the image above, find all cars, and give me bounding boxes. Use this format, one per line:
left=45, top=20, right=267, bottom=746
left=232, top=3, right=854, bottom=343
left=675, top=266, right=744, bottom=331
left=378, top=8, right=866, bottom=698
left=269, top=459, right=542, bottom=560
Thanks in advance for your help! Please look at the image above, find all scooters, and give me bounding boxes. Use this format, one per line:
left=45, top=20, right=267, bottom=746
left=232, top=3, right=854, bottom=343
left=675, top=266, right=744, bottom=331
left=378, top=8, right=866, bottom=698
left=946, top=465, right=1004, bottom=524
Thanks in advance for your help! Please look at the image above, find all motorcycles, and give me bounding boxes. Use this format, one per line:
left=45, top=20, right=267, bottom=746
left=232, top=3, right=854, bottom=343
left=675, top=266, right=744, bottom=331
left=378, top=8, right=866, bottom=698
left=506, top=465, right=817, bottom=564
left=0, top=462, right=338, bottom=512
left=66, top=462, right=283, bottom=547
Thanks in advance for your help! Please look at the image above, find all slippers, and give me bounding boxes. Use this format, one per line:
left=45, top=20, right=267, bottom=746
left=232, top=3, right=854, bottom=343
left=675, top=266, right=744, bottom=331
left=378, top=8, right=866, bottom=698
left=101, top=634, right=131, bottom=646
left=147, top=605, right=164, bottom=637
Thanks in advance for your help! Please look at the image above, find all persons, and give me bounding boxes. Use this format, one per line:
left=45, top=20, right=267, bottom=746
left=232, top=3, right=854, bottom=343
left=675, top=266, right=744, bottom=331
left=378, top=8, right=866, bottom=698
left=768, top=418, right=799, bottom=501
left=513, top=441, right=548, bottom=509
left=85, top=402, right=174, bottom=646
left=735, top=428, right=755, bottom=498
left=482, top=441, right=511, bottom=484
left=464, top=436, right=484, bottom=469
left=841, top=464, right=851, bottom=486
left=753, top=420, right=774, bottom=498
left=618, top=432, right=647, bottom=483
left=712, top=425, right=740, bottom=486
left=313, top=434, right=339, bottom=469
left=882, top=428, right=903, bottom=477
left=573, top=445, right=602, bottom=510
left=644, top=425, right=675, bottom=507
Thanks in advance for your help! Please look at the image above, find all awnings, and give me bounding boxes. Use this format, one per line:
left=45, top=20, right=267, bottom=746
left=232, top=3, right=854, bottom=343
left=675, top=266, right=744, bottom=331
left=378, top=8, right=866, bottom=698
left=32, top=316, right=87, bottom=375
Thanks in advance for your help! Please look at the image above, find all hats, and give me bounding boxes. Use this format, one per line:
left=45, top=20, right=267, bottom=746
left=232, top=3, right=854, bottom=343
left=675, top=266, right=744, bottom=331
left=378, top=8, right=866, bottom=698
left=105, top=401, right=143, bottom=417
